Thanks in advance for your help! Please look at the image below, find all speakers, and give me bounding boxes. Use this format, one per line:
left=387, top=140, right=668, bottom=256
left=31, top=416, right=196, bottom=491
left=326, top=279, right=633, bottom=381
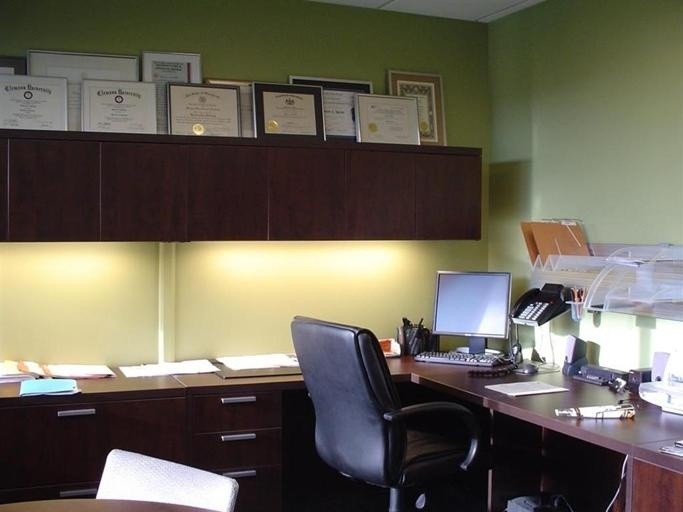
left=651, top=352, right=672, bottom=382
left=562, top=334, right=588, bottom=376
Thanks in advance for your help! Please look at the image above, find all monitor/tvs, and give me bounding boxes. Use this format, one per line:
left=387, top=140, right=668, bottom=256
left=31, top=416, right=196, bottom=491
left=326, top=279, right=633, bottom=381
left=431, top=270, right=512, bottom=354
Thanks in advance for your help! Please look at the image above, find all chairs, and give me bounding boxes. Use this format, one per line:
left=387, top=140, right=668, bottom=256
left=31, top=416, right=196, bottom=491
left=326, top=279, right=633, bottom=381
left=290, top=314, right=502, bottom=512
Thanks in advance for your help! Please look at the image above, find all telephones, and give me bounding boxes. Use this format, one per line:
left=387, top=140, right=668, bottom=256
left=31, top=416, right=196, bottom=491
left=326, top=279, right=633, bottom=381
left=510, top=283, right=571, bottom=326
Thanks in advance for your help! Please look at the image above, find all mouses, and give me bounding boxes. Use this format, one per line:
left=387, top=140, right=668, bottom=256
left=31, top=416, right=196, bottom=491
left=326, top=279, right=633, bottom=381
left=523, top=364, right=537, bottom=373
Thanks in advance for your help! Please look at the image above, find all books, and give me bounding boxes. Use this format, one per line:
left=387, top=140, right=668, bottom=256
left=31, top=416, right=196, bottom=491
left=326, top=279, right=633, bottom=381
left=0, top=359, right=118, bottom=398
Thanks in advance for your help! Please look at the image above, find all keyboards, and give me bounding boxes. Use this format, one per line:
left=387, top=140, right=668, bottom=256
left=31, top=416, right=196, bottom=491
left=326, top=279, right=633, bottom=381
left=414, top=351, right=503, bottom=366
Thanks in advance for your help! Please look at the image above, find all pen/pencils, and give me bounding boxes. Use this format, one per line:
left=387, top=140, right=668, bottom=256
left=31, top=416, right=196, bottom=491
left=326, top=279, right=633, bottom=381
left=403, top=317, right=423, bottom=355
left=571, top=286, right=586, bottom=320
